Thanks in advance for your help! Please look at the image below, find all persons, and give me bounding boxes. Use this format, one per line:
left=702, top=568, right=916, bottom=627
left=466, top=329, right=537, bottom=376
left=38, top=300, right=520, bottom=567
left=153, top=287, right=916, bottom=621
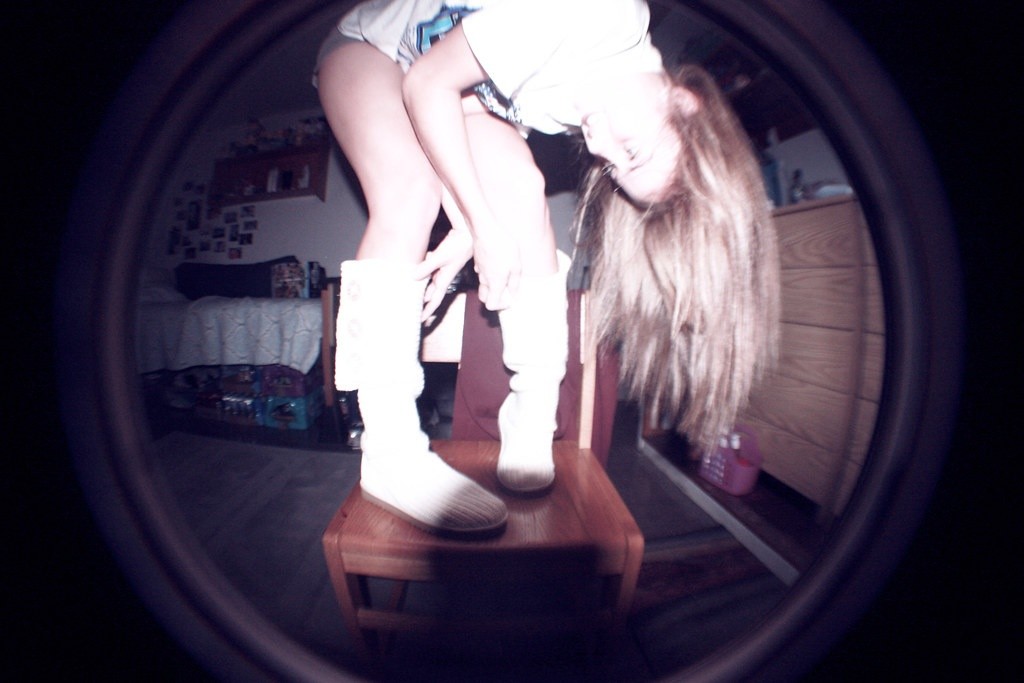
left=311, top=0, right=783, bottom=540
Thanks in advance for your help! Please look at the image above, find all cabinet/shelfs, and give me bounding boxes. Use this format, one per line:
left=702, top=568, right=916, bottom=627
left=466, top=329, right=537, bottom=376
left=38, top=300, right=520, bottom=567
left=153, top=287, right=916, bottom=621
left=699, top=189, right=888, bottom=529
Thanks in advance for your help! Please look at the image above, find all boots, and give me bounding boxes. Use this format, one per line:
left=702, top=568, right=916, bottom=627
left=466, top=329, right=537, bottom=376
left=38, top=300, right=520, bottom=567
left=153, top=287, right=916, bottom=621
left=469, top=250, right=573, bottom=499
left=337, top=257, right=508, bottom=538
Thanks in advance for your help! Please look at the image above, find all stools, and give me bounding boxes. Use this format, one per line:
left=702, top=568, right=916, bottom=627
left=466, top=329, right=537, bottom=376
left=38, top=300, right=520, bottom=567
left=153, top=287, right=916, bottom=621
left=323, top=440, right=645, bottom=680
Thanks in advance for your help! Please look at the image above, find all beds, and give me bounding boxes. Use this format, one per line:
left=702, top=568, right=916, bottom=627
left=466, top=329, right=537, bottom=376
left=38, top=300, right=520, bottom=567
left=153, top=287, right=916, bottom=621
left=132, top=281, right=356, bottom=449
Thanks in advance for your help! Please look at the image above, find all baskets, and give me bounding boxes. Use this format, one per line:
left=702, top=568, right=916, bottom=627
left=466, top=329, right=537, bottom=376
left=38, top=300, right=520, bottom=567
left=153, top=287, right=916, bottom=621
left=699, top=424, right=763, bottom=497
